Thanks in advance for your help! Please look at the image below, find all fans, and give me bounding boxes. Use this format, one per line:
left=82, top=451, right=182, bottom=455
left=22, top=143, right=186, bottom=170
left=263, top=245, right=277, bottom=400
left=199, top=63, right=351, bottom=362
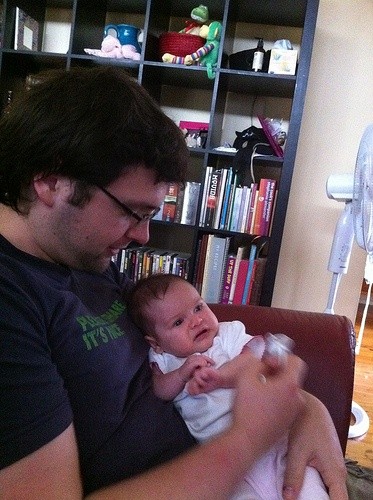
left=324, top=123, right=373, bottom=442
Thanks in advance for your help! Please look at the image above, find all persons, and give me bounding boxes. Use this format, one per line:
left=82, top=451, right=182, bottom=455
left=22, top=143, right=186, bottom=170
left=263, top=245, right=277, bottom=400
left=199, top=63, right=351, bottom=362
left=0, top=68, right=351, bottom=500
left=184, top=132, right=202, bottom=148
left=124, top=272, right=330, bottom=500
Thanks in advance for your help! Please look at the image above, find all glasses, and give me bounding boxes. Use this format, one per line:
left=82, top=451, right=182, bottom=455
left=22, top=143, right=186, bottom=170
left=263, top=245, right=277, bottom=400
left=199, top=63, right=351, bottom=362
left=95, top=185, right=160, bottom=224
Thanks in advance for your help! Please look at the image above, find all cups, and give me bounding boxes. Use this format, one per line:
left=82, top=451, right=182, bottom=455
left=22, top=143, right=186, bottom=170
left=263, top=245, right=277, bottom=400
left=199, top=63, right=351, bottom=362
left=264, top=117, right=281, bottom=136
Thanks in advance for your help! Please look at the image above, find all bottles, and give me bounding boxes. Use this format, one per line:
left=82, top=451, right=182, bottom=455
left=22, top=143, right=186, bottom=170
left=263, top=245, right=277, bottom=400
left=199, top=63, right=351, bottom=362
left=2, top=90, right=14, bottom=114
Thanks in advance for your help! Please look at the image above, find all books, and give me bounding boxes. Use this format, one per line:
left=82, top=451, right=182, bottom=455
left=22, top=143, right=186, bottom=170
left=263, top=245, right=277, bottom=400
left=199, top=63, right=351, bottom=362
left=111, top=165, right=279, bottom=306
left=14, top=8, right=39, bottom=51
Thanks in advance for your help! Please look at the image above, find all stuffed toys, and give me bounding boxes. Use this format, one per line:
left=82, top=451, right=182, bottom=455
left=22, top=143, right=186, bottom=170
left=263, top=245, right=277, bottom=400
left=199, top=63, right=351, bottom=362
left=83, top=35, right=141, bottom=60
left=163, top=4, right=222, bottom=80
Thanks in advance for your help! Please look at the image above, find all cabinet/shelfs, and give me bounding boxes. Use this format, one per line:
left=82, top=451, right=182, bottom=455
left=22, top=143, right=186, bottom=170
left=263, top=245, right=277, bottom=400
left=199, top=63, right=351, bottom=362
left=0, top=0, right=319, bottom=309
